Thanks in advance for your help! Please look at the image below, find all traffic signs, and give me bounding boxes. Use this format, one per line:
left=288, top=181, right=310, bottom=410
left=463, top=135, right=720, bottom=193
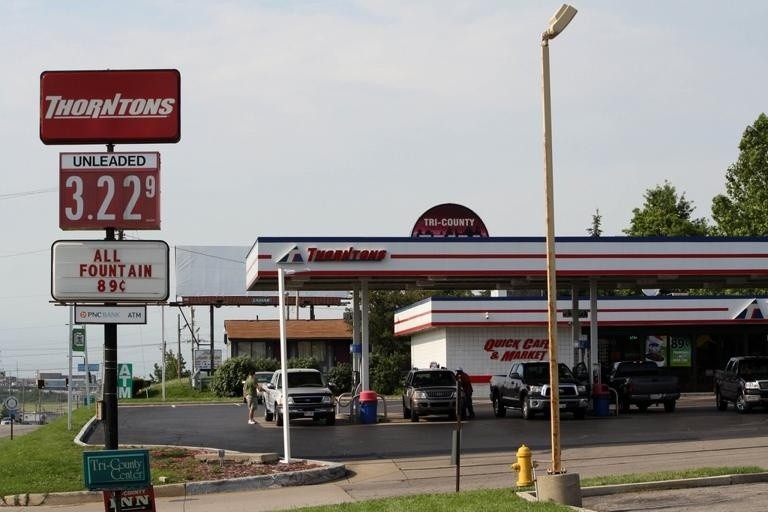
left=78, top=363, right=100, bottom=371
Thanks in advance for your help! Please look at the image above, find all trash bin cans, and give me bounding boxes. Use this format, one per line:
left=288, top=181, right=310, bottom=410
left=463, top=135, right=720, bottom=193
left=591, top=382, right=611, bottom=417
left=357, top=390, right=379, bottom=424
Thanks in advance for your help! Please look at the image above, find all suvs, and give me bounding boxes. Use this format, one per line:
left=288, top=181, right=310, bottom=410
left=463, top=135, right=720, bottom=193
left=402, top=365, right=467, bottom=421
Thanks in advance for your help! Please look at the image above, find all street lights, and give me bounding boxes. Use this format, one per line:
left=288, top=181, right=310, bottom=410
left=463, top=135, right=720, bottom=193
left=543, top=5, right=579, bottom=472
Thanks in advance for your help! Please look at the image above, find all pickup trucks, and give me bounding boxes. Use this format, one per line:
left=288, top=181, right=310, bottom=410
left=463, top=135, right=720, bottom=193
left=712, top=355, right=767, bottom=413
left=605, top=361, right=681, bottom=413
left=489, top=360, right=589, bottom=419
left=261, top=367, right=336, bottom=425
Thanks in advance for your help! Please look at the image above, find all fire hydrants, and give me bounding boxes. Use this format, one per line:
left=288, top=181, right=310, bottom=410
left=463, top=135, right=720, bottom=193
left=511, top=446, right=537, bottom=487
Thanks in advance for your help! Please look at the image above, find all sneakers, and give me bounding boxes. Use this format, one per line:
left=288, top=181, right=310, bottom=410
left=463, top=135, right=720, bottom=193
left=248, top=420, right=256, bottom=424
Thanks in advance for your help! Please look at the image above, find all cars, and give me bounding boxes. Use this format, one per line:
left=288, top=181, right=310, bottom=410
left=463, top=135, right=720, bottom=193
left=242, top=372, right=274, bottom=403
left=1, top=417, right=14, bottom=425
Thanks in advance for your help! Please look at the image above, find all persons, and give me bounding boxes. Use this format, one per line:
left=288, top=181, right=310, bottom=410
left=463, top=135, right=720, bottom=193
left=454, top=367, right=475, bottom=420
left=243, top=370, right=266, bottom=425
